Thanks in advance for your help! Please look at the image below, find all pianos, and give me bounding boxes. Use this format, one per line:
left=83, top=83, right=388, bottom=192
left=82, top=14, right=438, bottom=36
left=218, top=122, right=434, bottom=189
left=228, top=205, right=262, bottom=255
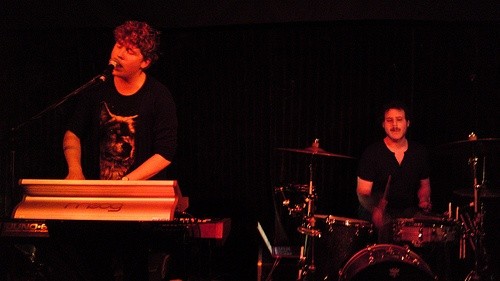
left=0, top=179, right=233, bottom=281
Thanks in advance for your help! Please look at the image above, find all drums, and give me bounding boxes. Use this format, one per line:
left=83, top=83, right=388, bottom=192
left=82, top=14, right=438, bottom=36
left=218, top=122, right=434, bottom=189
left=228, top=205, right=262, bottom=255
left=394, top=216, right=455, bottom=246
left=338, top=245, right=438, bottom=280
left=272, top=180, right=320, bottom=217
left=308, top=212, right=377, bottom=274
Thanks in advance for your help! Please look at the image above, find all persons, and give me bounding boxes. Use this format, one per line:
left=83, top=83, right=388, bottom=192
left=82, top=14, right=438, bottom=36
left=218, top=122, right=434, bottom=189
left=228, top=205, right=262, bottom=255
left=356, top=103, right=433, bottom=245
left=62, top=20, right=178, bottom=280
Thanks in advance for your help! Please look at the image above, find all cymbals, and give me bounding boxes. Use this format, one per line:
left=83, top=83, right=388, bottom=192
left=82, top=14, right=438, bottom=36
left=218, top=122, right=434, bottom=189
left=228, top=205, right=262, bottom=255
left=268, top=141, right=352, bottom=160
left=443, top=139, right=497, bottom=152
left=459, top=185, right=496, bottom=199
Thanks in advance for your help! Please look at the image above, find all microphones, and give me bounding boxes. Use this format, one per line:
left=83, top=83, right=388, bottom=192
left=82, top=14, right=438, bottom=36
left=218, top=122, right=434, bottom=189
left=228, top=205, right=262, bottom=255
left=99, top=59, right=118, bottom=83
left=443, top=202, right=474, bottom=216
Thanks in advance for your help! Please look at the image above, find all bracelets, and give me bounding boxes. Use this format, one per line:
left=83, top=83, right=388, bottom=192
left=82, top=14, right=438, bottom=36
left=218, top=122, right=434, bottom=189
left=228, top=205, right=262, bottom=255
left=125, top=176, right=130, bottom=180
